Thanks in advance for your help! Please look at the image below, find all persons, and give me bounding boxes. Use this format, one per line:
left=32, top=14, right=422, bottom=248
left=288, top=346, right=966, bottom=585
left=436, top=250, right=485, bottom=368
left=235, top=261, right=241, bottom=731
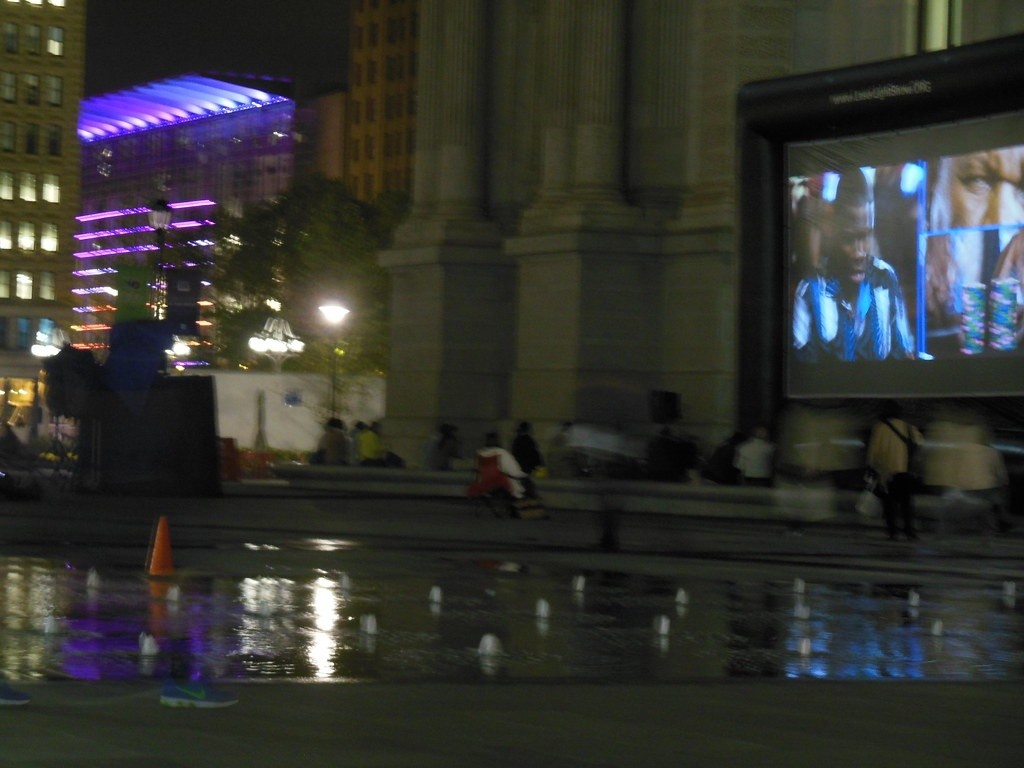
left=792, top=146, right=1024, bottom=361
left=863, top=406, right=925, bottom=542
left=317, top=418, right=391, bottom=468
left=473, top=420, right=547, bottom=509
left=735, top=427, right=777, bottom=487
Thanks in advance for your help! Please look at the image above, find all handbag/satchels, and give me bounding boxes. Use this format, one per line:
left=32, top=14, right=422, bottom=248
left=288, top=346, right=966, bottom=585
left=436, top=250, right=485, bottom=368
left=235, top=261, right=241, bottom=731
left=907, top=440, right=919, bottom=473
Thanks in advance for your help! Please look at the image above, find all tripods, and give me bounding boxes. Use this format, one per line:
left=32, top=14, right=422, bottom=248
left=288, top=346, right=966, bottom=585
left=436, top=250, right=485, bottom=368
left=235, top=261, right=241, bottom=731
left=33, top=414, right=76, bottom=480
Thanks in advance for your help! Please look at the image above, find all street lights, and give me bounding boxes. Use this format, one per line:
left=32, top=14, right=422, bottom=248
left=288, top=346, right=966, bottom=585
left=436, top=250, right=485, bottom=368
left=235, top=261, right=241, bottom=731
left=316, top=298, right=353, bottom=417
left=149, top=200, right=175, bottom=318
left=147, top=516, right=185, bottom=581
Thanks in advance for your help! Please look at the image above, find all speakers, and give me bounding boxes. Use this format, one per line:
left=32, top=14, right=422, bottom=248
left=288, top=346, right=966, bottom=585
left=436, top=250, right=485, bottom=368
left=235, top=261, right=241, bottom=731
left=648, top=392, right=682, bottom=425
left=46, top=345, right=96, bottom=417
